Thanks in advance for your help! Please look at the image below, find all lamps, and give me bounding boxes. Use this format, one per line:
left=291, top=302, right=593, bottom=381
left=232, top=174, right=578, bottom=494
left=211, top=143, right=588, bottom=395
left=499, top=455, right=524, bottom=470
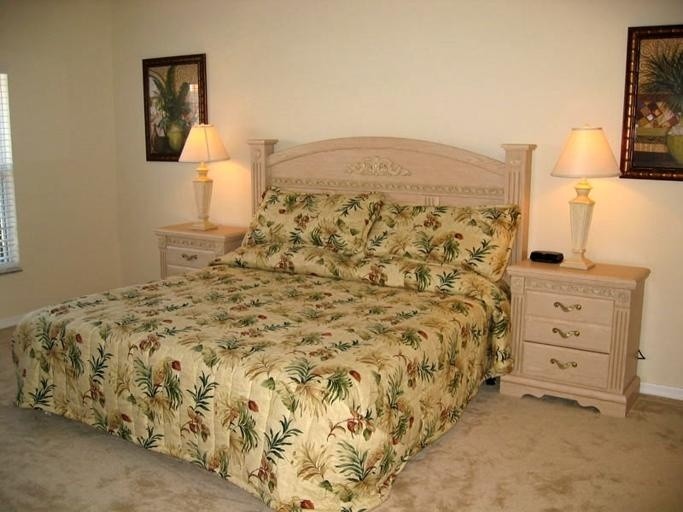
left=550, top=127, right=622, bottom=269
left=180, top=124, right=230, bottom=231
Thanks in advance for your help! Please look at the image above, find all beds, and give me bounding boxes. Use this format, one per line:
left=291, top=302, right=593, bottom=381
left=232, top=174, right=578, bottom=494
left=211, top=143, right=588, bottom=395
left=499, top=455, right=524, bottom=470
left=10, top=139, right=535, bottom=512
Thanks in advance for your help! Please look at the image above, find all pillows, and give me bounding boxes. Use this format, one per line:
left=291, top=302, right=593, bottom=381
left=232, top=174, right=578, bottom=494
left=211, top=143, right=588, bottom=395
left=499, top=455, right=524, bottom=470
left=241, top=185, right=384, bottom=255
left=358, top=198, right=520, bottom=280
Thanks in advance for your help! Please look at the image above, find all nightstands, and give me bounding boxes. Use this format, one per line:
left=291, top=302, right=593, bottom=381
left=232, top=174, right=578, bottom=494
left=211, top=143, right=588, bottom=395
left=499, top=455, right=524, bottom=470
left=154, top=222, right=247, bottom=280
left=496, top=262, right=650, bottom=418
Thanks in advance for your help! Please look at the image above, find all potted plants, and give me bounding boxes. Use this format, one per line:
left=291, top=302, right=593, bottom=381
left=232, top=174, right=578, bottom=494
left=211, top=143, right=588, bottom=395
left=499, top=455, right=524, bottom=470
left=642, top=44, right=683, bottom=164
left=146, top=65, right=191, bottom=151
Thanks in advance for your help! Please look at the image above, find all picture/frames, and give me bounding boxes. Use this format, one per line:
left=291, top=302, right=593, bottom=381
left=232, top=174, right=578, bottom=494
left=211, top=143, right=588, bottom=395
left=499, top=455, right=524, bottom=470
left=141, top=53, right=206, bottom=162
left=617, top=24, right=683, bottom=181
